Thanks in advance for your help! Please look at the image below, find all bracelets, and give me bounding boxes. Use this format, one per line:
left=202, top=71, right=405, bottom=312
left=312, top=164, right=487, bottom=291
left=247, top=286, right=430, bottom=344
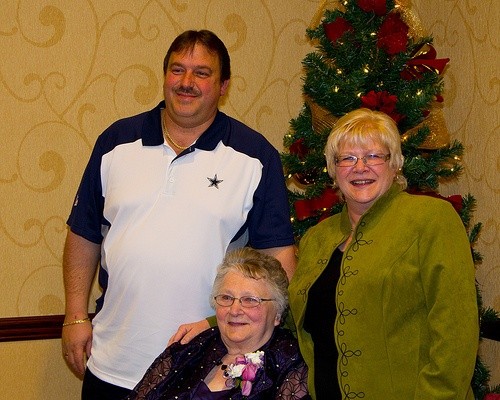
left=62, top=318, right=91, bottom=326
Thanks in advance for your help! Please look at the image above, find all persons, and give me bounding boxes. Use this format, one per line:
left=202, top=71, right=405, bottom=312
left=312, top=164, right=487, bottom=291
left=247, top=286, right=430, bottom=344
left=123, top=247, right=312, bottom=400
left=62, top=29, right=297, bottom=400
left=167, top=107, right=478, bottom=400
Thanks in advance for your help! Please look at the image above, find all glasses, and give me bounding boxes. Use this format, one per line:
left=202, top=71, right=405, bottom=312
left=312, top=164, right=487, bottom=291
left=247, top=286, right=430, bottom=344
left=215, top=291, right=281, bottom=309
left=333, top=152, right=397, bottom=169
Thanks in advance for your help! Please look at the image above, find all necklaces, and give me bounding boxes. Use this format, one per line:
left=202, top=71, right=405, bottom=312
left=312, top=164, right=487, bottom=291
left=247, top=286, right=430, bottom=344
left=218, top=362, right=228, bottom=377
left=163, top=114, right=189, bottom=152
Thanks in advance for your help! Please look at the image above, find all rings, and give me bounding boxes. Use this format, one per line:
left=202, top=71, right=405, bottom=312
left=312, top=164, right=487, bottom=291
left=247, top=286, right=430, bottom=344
left=64, top=352, right=69, bottom=357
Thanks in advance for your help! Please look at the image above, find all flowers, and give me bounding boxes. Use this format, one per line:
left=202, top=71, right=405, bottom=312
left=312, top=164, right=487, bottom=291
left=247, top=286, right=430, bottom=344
left=222, top=350, right=264, bottom=397
